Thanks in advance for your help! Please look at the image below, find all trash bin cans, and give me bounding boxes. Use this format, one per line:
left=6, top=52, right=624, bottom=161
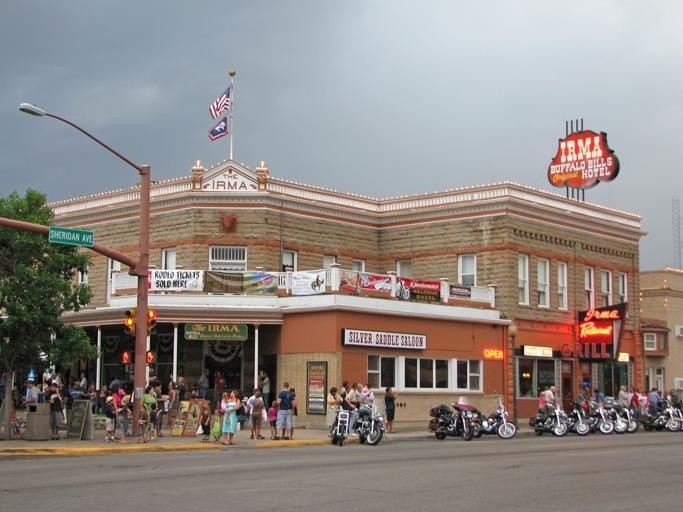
left=27, top=403, right=52, bottom=440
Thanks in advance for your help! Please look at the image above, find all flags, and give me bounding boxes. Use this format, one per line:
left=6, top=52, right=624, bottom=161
left=206, top=117, right=229, bottom=142
left=207, top=86, right=229, bottom=119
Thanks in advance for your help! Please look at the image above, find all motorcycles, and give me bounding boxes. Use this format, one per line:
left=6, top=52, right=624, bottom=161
left=355, top=400, right=387, bottom=445
left=469, top=388, right=519, bottom=440
left=528, top=402, right=568, bottom=437
left=427, top=402, right=480, bottom=440
left=328, top=406, right=353, bottom=447
left=563, top=400, right=682, bottom=435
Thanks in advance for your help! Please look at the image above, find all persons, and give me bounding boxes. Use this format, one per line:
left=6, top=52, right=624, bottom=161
left=382, top=385, right=401, bottom=434
left=535, top=382, right=676, bottom=415
left=66, top=371, right=134, bottom=441
left=23, top=368, right=65, bottom=440
left=139, top=377, right=185, bottom=442
left=327, top=381, right=373, bottom=435
left=268, top=382, right=298, bottom=439
left=198, top=367, right=267, bottom=443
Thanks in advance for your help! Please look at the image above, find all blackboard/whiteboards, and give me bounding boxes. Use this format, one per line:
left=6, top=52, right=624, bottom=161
left=306, top=361, right=328, bottom=415
left=68, top=401, right=87, bottom=433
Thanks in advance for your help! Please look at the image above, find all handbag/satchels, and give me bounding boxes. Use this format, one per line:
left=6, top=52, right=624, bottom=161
left=237, top=405, right=245, bottom=416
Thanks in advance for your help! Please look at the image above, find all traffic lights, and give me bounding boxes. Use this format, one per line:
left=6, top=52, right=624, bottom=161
left=144, top=350, right=155, bottom=365
left=121, top=350, right=130, bottom=364
left=146, top=308, right=158, bottom=336
left=124, top=308, right=136, bottom=335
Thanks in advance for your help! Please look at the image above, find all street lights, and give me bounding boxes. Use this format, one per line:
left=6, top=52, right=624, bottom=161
left=14, top=100, right=156, bottom=437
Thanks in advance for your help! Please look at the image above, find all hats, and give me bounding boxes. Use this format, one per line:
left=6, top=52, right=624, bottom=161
left=362, top=388, right=368, bottom=396
left=242, top=397, right=248, bottom=403
left=106, top=396, right=114, bottom=404
left=52, top=383, right=59, bottom=388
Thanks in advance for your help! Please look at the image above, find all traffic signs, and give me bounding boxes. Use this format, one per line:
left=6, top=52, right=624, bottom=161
left=48, top=225, right=95, bottom=248
